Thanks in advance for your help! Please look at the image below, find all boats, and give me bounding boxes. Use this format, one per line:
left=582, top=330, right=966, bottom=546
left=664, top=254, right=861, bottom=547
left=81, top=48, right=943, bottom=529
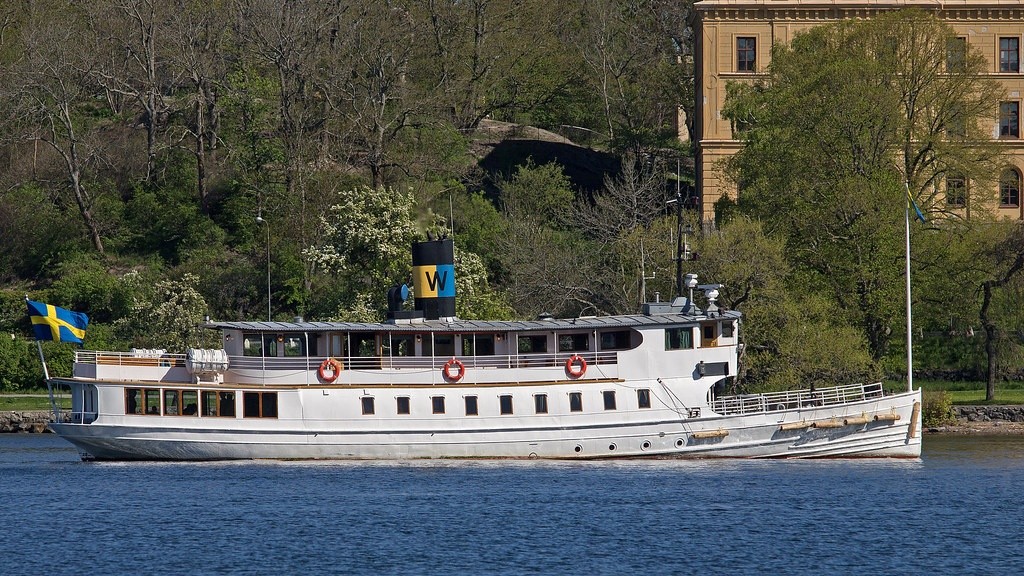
left=23, top=192, right=921, bottom=463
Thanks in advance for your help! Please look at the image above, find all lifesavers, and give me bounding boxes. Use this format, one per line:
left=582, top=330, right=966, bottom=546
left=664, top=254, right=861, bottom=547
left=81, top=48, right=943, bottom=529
left=444, top=360, right=465, bottom=380
left=319, top=360, right=340, bottom=382
left=567, top=356, right=587, bottom=376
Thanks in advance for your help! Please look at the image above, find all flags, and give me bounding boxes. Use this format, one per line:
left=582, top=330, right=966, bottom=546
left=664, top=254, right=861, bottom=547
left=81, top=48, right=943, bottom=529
left=907, top=188, right=926, bottom=222
left=27, top=301, right=88, bottom=344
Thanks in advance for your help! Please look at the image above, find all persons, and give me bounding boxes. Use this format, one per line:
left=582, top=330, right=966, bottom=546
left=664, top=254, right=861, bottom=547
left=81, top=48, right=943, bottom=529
left=126, top=390, right=137, bottom=413
left=149, top=406, right=159, bottom=414
left=220, top=392, right=234, bottom=416
